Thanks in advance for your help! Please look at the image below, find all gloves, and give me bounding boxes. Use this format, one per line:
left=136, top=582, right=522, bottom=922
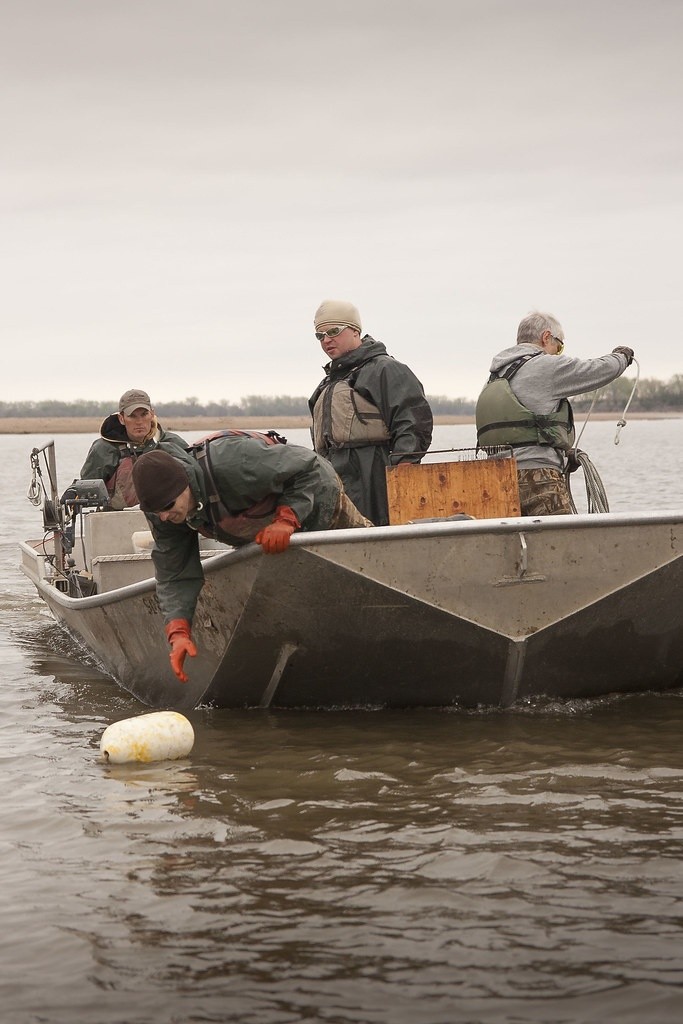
left=612, top=346, right=634, bottom=367
left=165, top=619, right=197, bottom=683
left=568, top=448, right=583, bottom=472
left=256, top=505, right=301, bottom=555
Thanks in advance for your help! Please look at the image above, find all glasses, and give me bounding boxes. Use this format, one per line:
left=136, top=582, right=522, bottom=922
left=551, top=335, right=564, bottom=355
left=315, top=326, right=348, bottom=340
left=161, top=501, right=176, bottom=511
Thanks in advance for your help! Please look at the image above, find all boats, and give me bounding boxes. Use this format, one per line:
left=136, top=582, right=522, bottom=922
left=18, top=437, right=682, bottom=718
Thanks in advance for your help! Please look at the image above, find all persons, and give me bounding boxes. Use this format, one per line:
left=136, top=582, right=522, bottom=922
left=131, top=429, right=376, bottom=682
left=308, top=300, right=433, bottom=526
left=475, top=312, right=634, bottom=516
left=80, top=388, right=190, bottom=511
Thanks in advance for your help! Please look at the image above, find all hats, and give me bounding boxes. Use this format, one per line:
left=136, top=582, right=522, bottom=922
left=132, top=450, right=190, bottom=512
left=314, top=300, right=362, bottom=333
left=119, top=389, right=151, bottom=416
